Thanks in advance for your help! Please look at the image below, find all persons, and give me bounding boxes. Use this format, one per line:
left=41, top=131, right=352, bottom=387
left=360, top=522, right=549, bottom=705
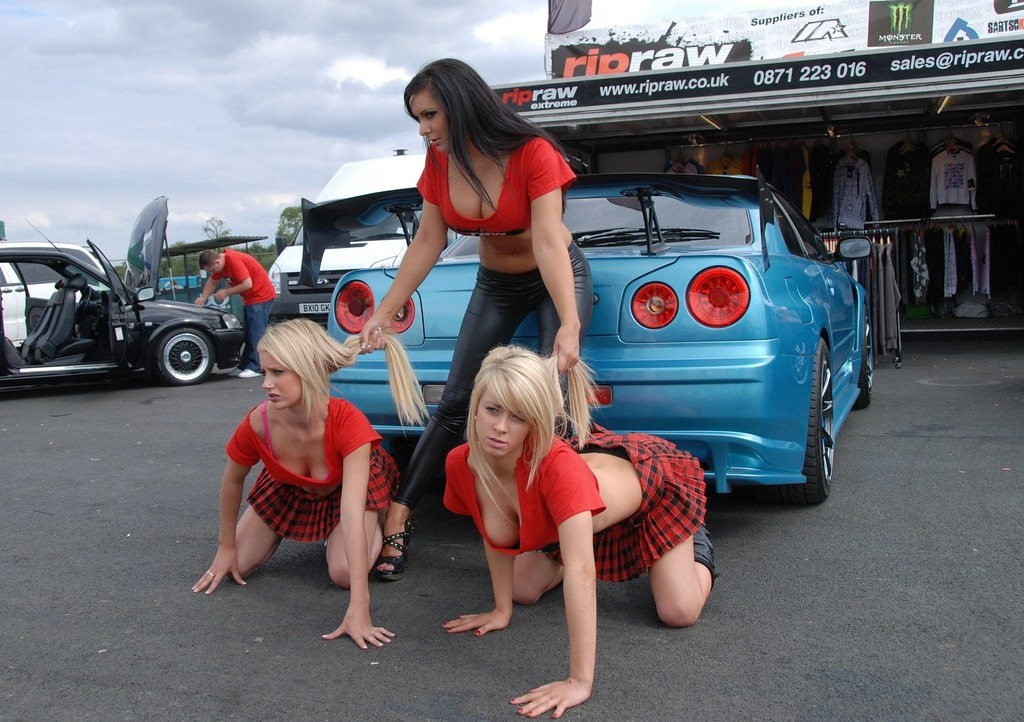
left=440, top=345, right=719, bottom=720
left=195, top=249, right=276, bottom=379
left=192, top=318, right=430, bottom=650
left=356, top=56, right=593, bottom=581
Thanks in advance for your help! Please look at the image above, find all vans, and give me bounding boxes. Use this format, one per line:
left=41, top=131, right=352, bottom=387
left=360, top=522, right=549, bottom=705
left=267, top=154, right=427, bottom=329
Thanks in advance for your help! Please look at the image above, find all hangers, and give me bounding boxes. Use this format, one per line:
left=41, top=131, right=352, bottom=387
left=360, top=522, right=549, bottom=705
left=674, top=130, right=1019, bottom=244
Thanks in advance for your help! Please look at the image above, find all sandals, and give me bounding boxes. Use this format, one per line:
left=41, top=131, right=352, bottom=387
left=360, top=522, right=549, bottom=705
left=376, top=510, right=415, bottom=579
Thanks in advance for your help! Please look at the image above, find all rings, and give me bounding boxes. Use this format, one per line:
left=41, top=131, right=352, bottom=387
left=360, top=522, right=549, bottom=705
left=567, top=367, right=573, bottom=373
left=207, top=570, right=213, bottom=577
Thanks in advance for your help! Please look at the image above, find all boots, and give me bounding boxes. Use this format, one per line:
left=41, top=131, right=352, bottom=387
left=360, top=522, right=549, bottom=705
left=693, top=520, right=715, bottom=590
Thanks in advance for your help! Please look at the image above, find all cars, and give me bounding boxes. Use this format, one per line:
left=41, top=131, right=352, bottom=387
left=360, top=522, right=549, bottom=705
left=298, top=174, right=876, bottom=506
left=0, top=196, right=248, bottom=392
left=0, top=243, right=111, bottom=347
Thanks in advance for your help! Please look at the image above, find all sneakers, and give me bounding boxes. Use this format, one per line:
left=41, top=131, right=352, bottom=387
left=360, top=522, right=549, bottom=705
left=226, top=367, right=243, bottom=377
left=238, top=369, right=262, bottom=377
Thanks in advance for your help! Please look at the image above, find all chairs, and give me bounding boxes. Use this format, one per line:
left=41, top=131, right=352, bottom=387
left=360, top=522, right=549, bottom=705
left=20, top=273, right=103, bottom=363
